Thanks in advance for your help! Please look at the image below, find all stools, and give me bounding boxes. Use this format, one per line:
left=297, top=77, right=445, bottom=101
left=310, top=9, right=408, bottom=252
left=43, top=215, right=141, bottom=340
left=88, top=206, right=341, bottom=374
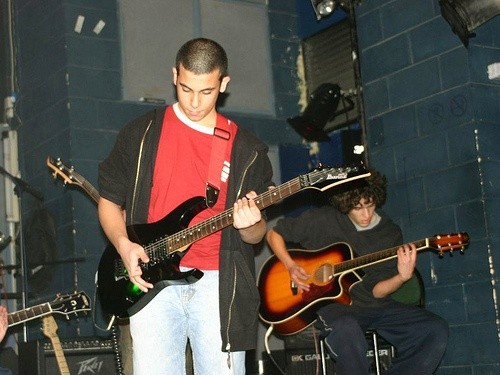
left=318, top=330, right=394, bottom=375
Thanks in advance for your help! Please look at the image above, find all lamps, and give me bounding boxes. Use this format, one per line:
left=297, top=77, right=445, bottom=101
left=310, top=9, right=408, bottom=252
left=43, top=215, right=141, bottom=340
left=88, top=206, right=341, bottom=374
left=311, top=0, right=339, bottom=21
left=433, top=0, right=500, bottom=49
left=286, top=83, right=354, bottom=144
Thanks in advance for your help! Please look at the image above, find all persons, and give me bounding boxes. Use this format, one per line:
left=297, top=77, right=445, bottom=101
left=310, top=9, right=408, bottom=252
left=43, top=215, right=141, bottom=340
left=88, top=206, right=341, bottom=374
left=97, top=37, right=273, bottom=375
left=266, top=167, right=450, bottom=374
left=0, top=305, right=9, bottom=343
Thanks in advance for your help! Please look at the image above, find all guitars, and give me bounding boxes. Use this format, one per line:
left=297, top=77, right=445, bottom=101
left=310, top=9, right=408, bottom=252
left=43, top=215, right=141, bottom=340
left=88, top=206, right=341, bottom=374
left=47, top=154, right=100, bottom=204
left=8, top=290, right=92, bottom=327
left=39, top=315, right=70, bottom=375
left=98, top=154, right=371, bottom=318
left=257, top=231, right=470, bottom=336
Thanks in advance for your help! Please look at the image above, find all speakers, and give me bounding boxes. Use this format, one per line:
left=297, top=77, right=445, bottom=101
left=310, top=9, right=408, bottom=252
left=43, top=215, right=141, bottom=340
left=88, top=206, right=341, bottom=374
left=262, top=342, right=399, bottom=375
left=19, top=335, right=122, bottom=375
